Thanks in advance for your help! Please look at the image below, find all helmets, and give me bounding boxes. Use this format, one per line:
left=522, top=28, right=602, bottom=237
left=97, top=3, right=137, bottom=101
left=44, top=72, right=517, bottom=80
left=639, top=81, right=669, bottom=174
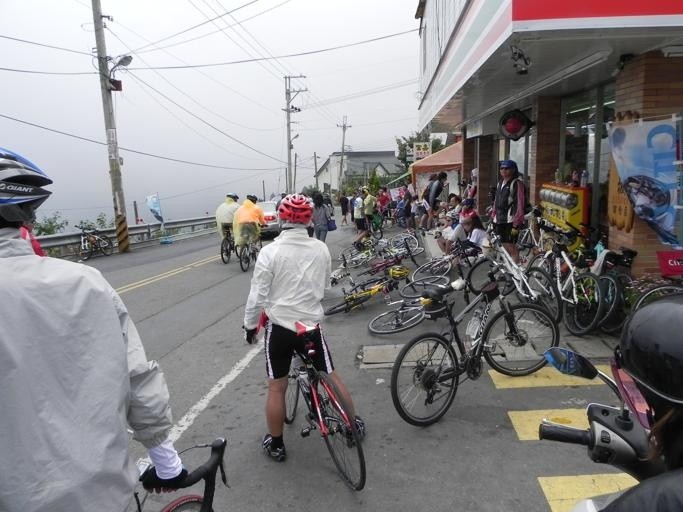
left=0, top=147, right=52, bottom=222
left=276, top=193, right=314, bottom=224
left=247, top=195, right=257, bottom=202
left=227, top=192, right=239, bottom=202
left=610, top=286, right=683, bottom=403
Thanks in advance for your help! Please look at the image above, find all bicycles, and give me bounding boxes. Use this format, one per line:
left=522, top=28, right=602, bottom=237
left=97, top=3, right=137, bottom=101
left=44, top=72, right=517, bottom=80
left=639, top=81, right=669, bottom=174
left=389, top=225, right=558, bottom=428
left=131, top=436, right=227, bottom=512
left=248, top=315, right=367, bottom=493
left=240, top=224, right=268, bottom=272
left=221, top=225, right=249, bottom=264
left=632, top=258, right=683, bottom=313
left=75, top=225, right=113, bottom=261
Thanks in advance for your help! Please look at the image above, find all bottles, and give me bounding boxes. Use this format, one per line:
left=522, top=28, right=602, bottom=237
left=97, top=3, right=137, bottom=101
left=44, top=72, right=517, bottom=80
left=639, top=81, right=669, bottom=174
left=464, top=305, right=483, bottom=341
left=297, top=365, right=311, bottom=393
left=553, top=167, right=562, bottom=187
left=571, top=171, right=578, bottom=187
left=579, top=169, right=588, bottom=188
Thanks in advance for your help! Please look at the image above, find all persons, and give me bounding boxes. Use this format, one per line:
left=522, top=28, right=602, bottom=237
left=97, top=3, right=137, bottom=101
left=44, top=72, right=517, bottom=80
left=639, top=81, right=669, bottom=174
left=0, top=147, right=187, bottom=512
left=312, top=159, right=527, bottom=263
left=217, top=192, right=266, bottom=254
left=242, top=195, right=366, bottom=462
left=596, top=295, right=682, bottom=512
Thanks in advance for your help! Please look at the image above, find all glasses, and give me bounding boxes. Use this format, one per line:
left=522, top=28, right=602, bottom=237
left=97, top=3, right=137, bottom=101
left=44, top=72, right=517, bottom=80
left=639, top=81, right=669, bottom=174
left=500, top=167, right=510, bottom=170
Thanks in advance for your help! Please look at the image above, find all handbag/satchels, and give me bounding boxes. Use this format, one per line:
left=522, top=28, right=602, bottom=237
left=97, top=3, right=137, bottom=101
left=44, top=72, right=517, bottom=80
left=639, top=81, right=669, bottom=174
left=327, top=217, right=337, bottom=231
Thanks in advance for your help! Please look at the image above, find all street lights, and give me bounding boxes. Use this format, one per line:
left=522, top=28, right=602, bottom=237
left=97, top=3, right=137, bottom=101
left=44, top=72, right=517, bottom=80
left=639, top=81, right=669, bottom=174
left=285, top=133, right=299, bottom=195
left=96, top=54, right=133, bottom=254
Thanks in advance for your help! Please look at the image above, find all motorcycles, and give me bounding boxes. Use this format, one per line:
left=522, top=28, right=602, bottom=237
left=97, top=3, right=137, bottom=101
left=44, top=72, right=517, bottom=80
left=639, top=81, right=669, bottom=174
left=538, top=346, right=668, bottom=511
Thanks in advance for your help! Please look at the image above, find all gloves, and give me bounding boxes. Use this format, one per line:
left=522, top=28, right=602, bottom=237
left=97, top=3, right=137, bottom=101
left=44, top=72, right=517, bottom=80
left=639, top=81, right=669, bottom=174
left=242, top=325, right=257, bottom=343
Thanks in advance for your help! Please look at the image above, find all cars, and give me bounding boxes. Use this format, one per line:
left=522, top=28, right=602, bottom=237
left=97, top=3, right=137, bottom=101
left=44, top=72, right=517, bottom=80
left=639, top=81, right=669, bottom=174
left=255, top=201, right=283, bottom=240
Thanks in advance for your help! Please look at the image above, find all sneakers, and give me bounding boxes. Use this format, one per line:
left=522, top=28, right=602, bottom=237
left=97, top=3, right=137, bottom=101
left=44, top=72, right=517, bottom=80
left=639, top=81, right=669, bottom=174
left=346, top=416, right=365, bottom=446
left=262, top=434, right=285, bottom=461
